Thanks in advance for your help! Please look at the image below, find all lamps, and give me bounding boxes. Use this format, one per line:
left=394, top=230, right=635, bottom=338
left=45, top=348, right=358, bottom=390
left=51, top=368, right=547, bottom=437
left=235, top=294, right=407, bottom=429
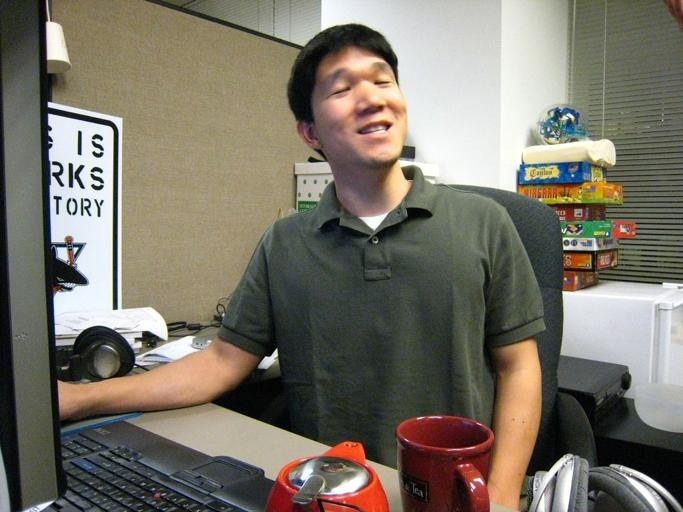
left=43, top=0, right=72, bottom=74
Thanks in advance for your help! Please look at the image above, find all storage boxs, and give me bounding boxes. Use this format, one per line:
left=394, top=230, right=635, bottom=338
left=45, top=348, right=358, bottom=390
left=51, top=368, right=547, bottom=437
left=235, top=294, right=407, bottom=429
left=288, top=158, right=439, bottom=214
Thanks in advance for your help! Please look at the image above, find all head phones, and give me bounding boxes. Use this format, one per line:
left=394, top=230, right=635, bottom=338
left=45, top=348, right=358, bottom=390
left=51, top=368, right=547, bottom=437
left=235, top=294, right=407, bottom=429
left=57, top=326, right=135, bottom=382
left=527, top=453, right=683, bottom=512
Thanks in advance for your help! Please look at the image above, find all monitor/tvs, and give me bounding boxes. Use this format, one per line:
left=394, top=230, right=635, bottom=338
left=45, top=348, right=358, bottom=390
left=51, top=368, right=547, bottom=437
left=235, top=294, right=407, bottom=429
left=0, top=1, right=67, bottom=512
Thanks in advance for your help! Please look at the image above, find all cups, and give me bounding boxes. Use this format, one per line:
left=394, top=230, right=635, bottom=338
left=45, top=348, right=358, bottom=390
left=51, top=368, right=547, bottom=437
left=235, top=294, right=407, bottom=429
left=394, top=415, right=495, bottom=512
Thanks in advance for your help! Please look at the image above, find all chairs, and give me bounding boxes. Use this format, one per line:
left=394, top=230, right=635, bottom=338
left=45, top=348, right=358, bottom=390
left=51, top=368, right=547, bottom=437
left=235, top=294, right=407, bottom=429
left=205, top=183, right=603, bottom=512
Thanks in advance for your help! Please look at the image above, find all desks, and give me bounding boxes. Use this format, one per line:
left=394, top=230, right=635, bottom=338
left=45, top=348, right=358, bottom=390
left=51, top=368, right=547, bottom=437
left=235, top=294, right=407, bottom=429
left=66, top=322, right=281, bottom=394
left=59, top=399, right=403, bottom=512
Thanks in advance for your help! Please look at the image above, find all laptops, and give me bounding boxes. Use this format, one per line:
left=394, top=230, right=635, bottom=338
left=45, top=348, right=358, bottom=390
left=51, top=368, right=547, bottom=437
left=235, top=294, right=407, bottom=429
left=0, top=417, right=275, bottom=512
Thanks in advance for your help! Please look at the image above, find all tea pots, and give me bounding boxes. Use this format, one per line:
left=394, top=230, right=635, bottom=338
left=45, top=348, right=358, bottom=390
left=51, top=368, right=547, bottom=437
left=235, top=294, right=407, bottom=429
left=263, top=439, right=391, bottom=512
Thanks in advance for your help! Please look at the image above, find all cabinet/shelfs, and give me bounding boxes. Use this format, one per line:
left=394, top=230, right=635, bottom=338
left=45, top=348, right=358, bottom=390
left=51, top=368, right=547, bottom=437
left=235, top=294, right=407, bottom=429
left=597, top=395, right=682, bottom=512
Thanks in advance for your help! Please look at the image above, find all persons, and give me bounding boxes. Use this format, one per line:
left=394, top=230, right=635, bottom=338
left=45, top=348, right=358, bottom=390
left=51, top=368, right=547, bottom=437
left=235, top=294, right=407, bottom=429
left=58, top=21, right=549, bottom=512
